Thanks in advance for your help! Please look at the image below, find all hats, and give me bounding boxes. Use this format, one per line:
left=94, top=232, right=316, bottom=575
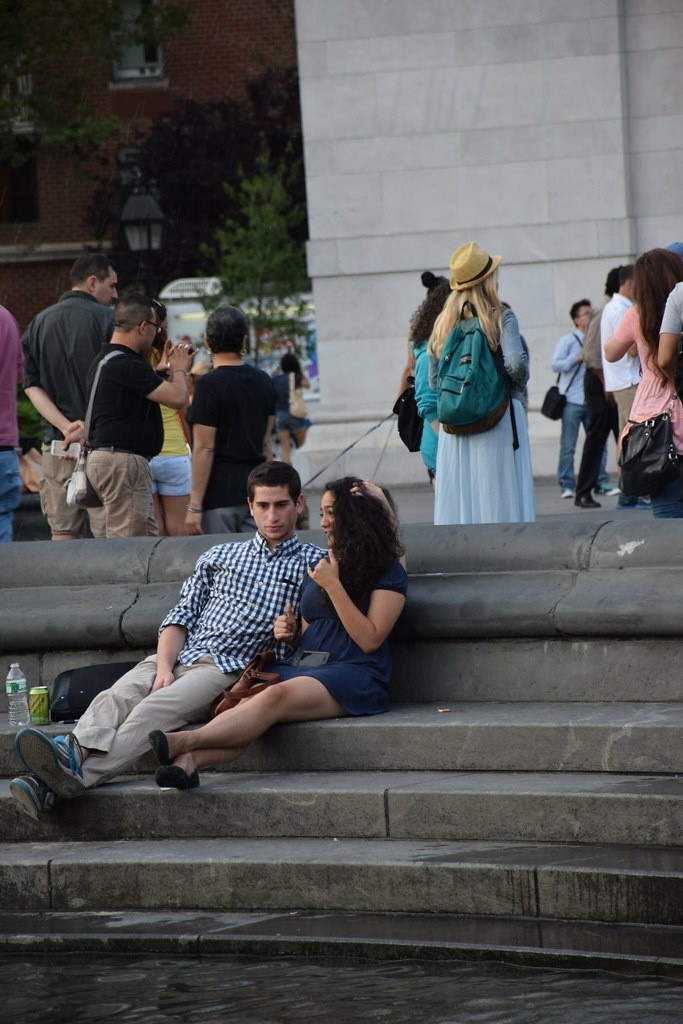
left=449, top=241, right=504, bottom=290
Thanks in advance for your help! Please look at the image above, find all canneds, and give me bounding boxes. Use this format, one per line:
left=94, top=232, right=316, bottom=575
left=28, top=686, right=50, bottom=724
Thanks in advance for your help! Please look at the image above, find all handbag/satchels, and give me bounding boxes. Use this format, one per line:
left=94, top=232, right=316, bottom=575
left=50, top=661, right=143, bottom=721
left=617, top=413, right=681, bottom=496
left=541, top=386, right=567, bottom=420
left=63, top=445, right=104, bottom=508
left=392, top=376, right=425, bottom=451
left=288, top=372, right=309, bottom=417
left=209, top=651, right=280, bottom=716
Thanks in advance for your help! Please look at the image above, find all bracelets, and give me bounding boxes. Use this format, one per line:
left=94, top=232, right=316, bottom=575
left=171, top=369, right=187, bottom=376
left=187, top=504, right=203, bottom=514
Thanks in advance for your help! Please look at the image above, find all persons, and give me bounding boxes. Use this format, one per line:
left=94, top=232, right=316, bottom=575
left=66, top=293, right=199, bottom=539
left=540, top=299, right=621, bottom=498
left=392, top=270, right=454, bottom=488
left=148, top=475, right=409, bottom=790
left=0, top=298, right=23, bottom=544
left=7, top=462, right=306, bottom=820
left=429, top=241, right=536, bottom=524
left=145, top=297, right=200, bottom=536
left=20, top=250, right=119, bottom=541
left=270, top=352, right=312, bottom=467
left=182, top=305, right=279, bottom=535
left=575, top=265, right=644, bottom=506
left=603, top=243, right=683, bottom=519
left=601, top=265, right=651, bottom=507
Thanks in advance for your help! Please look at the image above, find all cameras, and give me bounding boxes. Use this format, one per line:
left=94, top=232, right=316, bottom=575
left=179, top=343, right=197, bottom=355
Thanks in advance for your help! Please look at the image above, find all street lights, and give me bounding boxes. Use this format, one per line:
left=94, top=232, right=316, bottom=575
left=121, top=179, right=168, bottom=300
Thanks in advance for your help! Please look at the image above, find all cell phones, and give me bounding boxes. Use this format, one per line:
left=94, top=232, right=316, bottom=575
left=297, top=650, right=331, bottom=667
left=51, top=440, right=80, bottom=458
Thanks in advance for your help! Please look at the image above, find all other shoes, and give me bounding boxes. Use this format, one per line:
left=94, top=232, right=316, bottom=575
left=154, top=762, right=200, bottom=790
left=148, top=730, right=170, bottom=766
left=594, top=482, right=620, bottom=496
left=575, top=494, right=602, bottom=509
left=636, top=495, right=652, bottom=509
left=615, top=495, right=637, bottom=509
left=561, top=487, right=573, bottom=499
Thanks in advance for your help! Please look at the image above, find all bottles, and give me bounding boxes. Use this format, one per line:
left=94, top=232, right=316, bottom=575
left=6, top=663, right=30, bottom=726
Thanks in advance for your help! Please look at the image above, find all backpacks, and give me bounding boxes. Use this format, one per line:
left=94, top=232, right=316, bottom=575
left=436, top=300, right=512, bottom=436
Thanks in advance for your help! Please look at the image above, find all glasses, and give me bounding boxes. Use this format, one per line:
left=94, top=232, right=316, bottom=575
left=138, top=320, right=162, bottom=333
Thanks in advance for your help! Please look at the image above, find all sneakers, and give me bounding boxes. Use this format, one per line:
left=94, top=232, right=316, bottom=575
left=15, top=727, right=86, bottom=799
left=10, top=775, right=57, bottom=821
